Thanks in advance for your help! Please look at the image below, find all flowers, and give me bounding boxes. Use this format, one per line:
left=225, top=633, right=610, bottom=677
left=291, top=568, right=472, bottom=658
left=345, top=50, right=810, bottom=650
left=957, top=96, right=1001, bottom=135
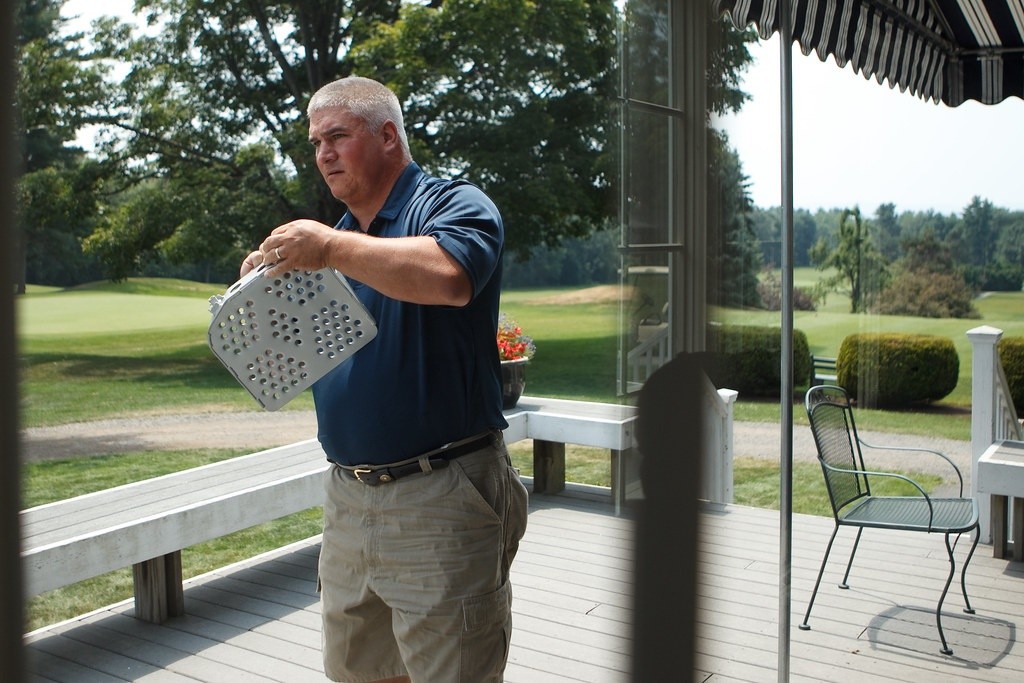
left=496, top=312, right=537, bottom=361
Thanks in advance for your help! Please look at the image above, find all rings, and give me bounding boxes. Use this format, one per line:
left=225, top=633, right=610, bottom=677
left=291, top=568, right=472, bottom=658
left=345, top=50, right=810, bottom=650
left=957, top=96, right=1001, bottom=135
left=275, top=247, right=282, bottom=259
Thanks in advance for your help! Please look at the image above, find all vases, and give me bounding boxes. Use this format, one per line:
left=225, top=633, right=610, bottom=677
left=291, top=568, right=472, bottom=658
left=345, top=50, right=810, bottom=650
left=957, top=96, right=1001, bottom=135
left=500, top=356, right=528, bottom=410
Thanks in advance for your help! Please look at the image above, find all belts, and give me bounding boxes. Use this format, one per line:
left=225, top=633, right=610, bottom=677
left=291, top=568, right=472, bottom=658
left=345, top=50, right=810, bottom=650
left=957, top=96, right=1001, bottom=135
left=326, top=431, right=504, bottom=486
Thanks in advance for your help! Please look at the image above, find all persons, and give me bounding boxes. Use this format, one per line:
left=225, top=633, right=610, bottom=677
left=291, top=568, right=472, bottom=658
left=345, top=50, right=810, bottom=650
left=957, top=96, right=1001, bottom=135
left=238, top=77, right=531, bottom=683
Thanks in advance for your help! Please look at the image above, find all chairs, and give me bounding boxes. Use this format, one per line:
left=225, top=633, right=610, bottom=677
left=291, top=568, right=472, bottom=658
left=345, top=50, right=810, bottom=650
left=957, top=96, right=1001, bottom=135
left=798, top=385, right=981, bottom=654
